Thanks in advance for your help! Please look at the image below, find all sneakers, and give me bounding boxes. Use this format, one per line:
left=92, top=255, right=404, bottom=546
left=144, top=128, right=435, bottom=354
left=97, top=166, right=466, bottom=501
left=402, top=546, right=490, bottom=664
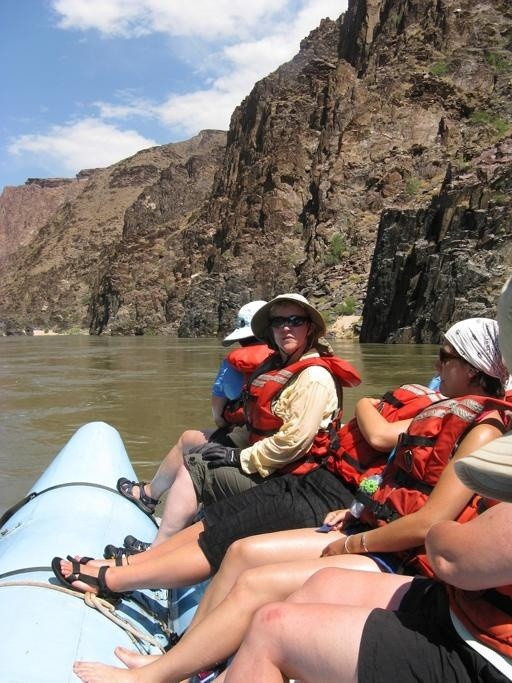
left=105, top=535, right=152, bottom=559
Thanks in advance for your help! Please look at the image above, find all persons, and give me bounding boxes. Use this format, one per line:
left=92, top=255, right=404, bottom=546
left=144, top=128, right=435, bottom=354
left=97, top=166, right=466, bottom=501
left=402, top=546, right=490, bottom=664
left=106, top=292, right=345, bottom=564
left=73, top=318, right=512, bottom=683
left=117, top=298, right=280, bottom=514
left=213, top=277, right=511, bottom=683
left=52, top=368, right=450, bottom=604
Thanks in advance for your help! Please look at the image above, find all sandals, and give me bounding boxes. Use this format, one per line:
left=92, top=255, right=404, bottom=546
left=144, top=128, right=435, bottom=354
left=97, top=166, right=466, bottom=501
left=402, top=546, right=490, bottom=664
left=117, top=477, right=161, bottom=515
left=51, top=555, right=123, bottom=604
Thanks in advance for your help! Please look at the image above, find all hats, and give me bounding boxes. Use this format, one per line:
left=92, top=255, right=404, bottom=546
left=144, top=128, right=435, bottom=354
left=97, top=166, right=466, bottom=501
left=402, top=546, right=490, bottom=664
left=222, top=299, right=268, bottom=348
left=252, top=293, right=327, bottom=346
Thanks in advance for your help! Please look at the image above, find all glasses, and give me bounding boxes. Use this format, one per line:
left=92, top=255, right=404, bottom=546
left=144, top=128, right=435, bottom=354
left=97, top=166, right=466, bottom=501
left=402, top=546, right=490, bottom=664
left=439, top=347, right=459, bottom=361
left=264, top=314, right=309, bottom=328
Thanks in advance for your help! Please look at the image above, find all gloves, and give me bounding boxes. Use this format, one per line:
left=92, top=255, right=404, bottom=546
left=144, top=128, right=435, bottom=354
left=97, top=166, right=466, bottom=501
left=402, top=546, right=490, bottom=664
left=202, top=445, right=241, bottom=469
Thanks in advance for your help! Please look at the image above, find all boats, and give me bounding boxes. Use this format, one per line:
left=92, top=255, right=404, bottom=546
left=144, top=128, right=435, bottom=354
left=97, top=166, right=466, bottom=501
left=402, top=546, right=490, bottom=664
left=0, top=419, right=217, bottom=682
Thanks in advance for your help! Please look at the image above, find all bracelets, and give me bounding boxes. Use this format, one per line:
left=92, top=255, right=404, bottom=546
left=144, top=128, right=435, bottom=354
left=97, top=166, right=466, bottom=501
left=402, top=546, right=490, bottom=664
left=345, top=534, right=353, bottom=555
left=361, top=531, right=369, bottom=553
